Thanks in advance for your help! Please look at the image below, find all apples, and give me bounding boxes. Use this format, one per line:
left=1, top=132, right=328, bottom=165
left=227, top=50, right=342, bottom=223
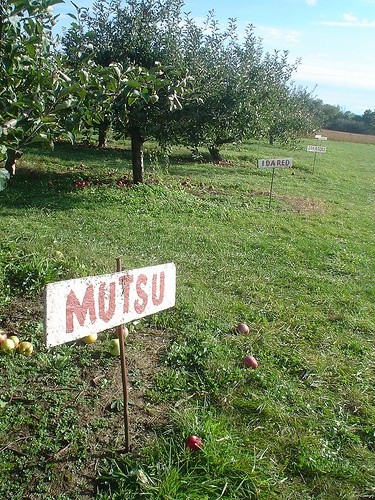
left=19, top=342, right=33, bottom=356
left=187, top=435, right=202, bottom=450
left=206, top=159, right=233, bottom=165
left=238, top=322, right=249, bottom=335
left=2, top=340, right=15, bottom=353
left=73, top=170, right=215, bottom=190
left=117, top=327, right=129, bottom=337
left=84, top=334, right=97, bottom=343
left=243, top=355, right=258, bottom=368
left=9, top=336, right=20, bottom=346
left=0, top=332, right=7, bottom=345
left=110, top=338, right=121, bottom=356
